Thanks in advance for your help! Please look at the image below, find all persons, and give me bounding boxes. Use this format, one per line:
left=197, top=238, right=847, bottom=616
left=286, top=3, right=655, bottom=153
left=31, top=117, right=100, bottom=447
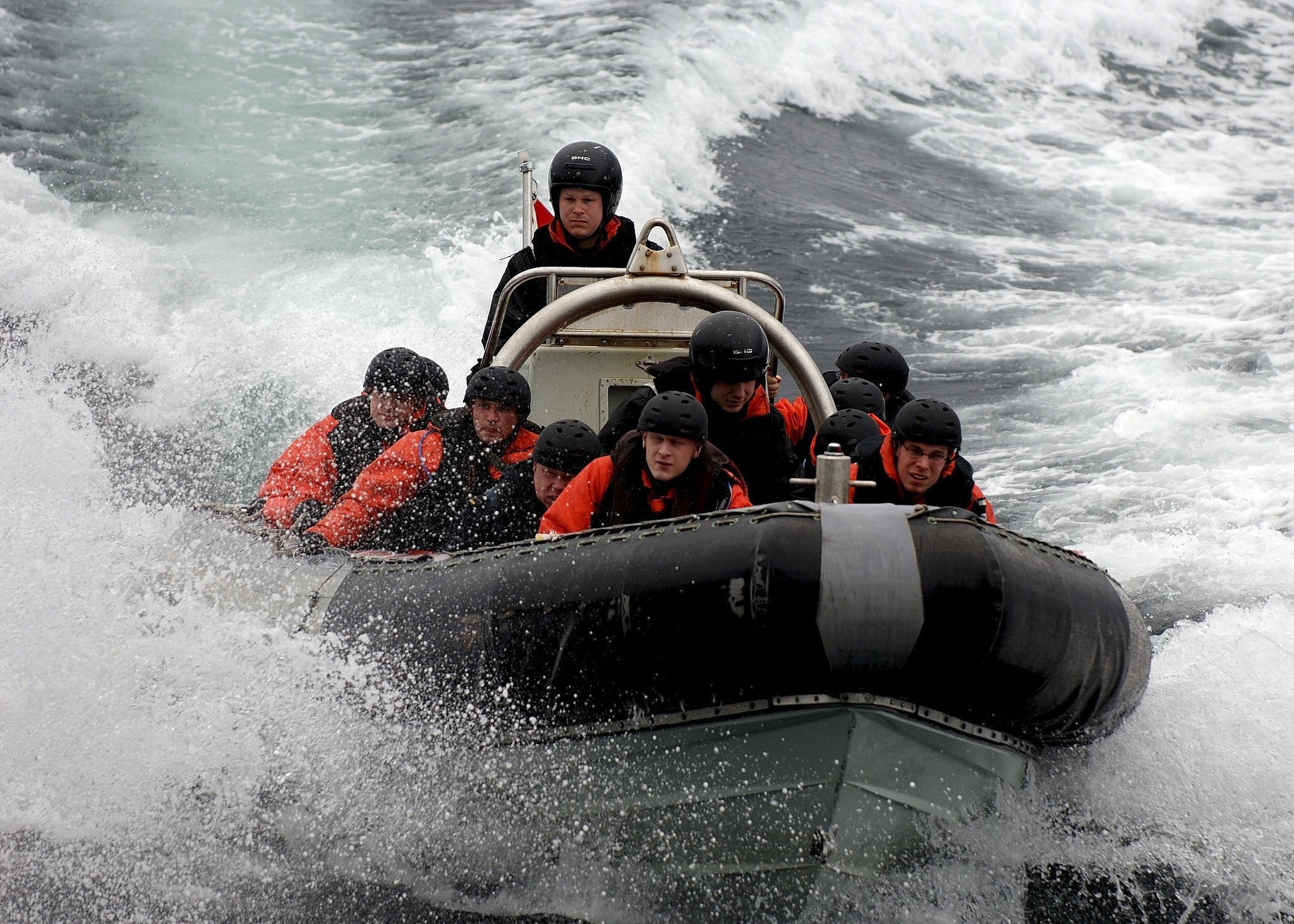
left=248, top=143, right=998, bottom=555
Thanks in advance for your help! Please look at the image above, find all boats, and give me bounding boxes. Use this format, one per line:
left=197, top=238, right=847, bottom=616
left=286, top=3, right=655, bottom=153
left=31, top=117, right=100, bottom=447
left=264, top=152, right=1154, bottom=924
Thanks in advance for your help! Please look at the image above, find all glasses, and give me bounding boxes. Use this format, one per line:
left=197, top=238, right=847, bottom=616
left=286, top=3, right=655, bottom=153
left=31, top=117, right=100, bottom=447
left=901, top=439, right=953, bottom=464
left=836, top=372, right=845, bottom=381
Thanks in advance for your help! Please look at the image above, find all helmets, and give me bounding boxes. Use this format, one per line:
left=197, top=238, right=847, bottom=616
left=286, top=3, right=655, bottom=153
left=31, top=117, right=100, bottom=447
left=548, top=141, right=623, bottom=223
left=363, top=346, right=449, bottom=408
left=463, top=366, right=531, bottom=418
left=688, top=310, right=769, bottom=396
left=636, top=391, right=708, bottom=447
left=835, top=341, right=909, bottom=404
left=814, top=409, right=881, bottom=462
left=891, top=398, right=962, bottom=453
left=532, top=419, right=602, bottom=473
left=831, top=376, right=887, bottom=422
